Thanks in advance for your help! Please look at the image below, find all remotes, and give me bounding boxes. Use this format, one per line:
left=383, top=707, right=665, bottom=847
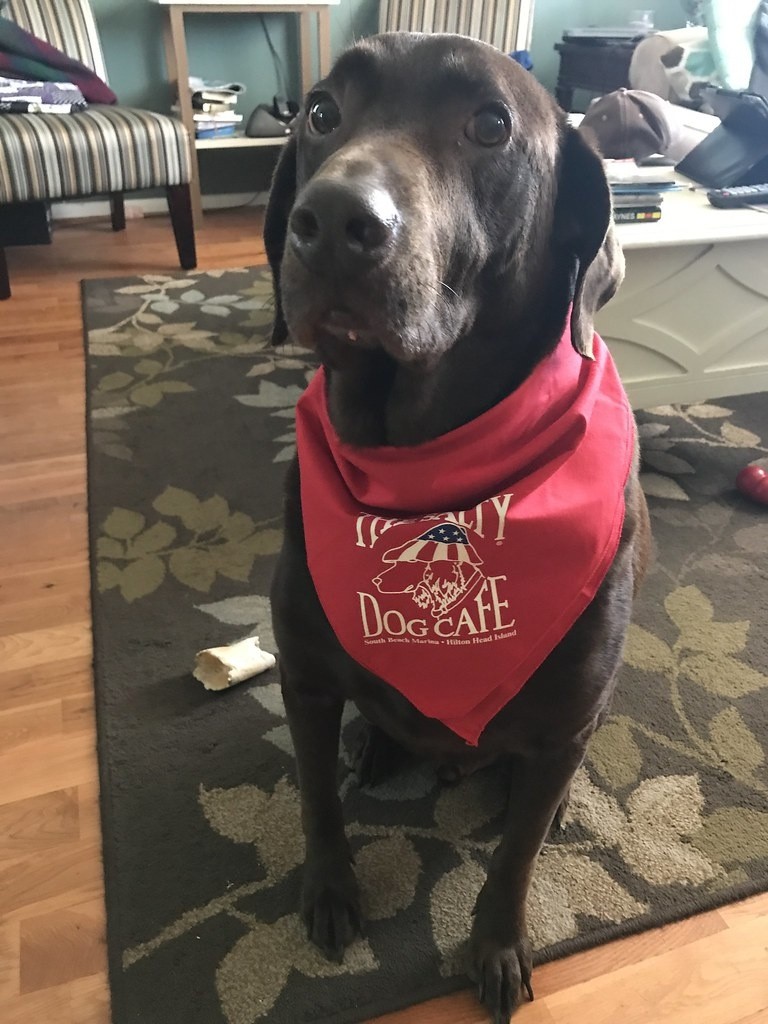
left=706, top=182, right=768, bottom=209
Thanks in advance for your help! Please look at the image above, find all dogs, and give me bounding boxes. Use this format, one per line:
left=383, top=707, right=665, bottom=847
left=262, top=31, right=653, bottom=1024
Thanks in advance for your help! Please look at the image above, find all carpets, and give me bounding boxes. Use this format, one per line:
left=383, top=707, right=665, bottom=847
left=79, top=266, right=768, bottom=1024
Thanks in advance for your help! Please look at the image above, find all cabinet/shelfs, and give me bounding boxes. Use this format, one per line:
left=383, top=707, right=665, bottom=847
left=159, top=0, right=333, bottom=231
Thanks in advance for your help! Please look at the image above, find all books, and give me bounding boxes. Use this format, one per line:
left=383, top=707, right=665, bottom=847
left=602, top=157, right=692, bottom=224
left=169, top=75, right=246, bottom=139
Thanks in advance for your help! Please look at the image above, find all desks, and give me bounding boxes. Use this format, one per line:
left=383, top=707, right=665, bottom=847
left=551, top=41, right=642, bottom=113
left=557, top=163, right=766, bottom=408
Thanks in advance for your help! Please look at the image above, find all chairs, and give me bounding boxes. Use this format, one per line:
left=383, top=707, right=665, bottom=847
left=0, top=0, right=198, bottom=302
left=380, top=0, right=535, bottom=75
